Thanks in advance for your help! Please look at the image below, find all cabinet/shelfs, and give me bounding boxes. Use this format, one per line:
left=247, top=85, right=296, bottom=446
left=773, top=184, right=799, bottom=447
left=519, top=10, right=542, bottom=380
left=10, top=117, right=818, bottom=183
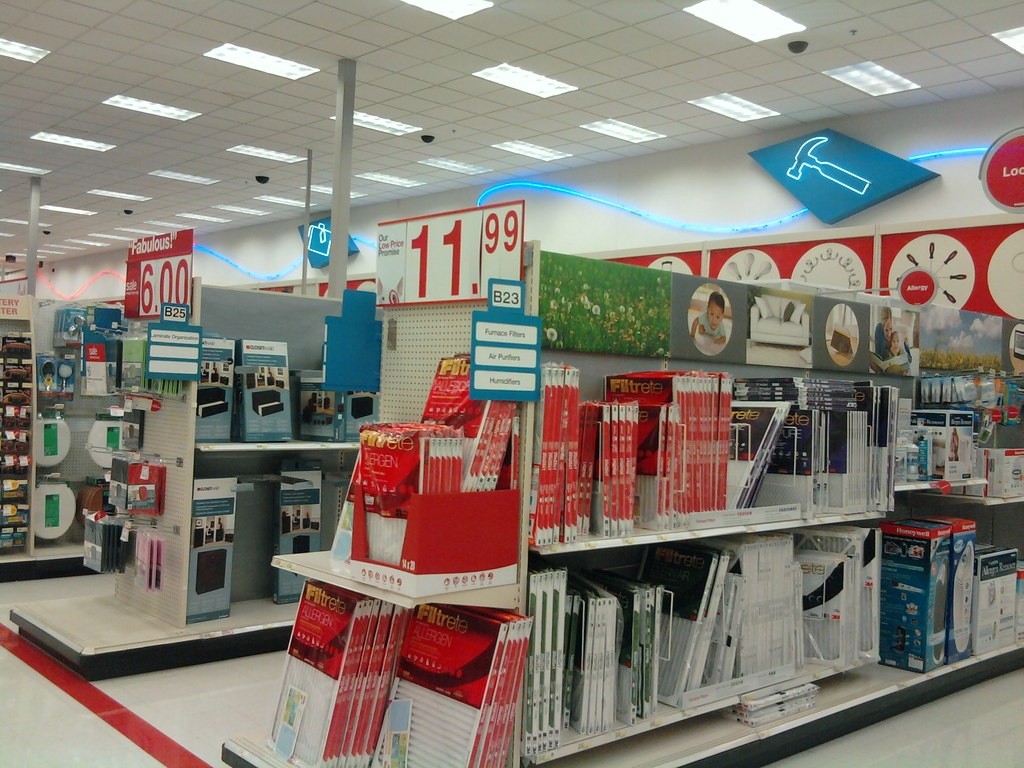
left=525, top=238, right=1023, bottom=767
left=223, top=236, right=526, bottom=768
left=7, top=277, right=382, bottom=683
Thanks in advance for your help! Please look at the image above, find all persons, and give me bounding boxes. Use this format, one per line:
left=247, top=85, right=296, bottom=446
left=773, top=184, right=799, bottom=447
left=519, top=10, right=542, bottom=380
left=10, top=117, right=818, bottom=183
left=874, top=317, right=913, bottom=376
left=691, top=291, right=727, bottom=345
left=948, top=428, right=960, bottom=461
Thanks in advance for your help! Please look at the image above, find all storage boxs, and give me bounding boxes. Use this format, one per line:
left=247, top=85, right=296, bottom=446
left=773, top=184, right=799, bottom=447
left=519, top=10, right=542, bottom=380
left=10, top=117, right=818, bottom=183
left=878, top=516, right=1023, bottom=763
left=195, top=335, right=235, bottom=445
left=186, top=471, right=237, bottom=623
left=897, top=362, right=1023, bottom=545
left=298, top=377, right=381, bottom=443
left=237, top=338, right=294, bottom=442
left=265, top=456, right=320, bottom=605
